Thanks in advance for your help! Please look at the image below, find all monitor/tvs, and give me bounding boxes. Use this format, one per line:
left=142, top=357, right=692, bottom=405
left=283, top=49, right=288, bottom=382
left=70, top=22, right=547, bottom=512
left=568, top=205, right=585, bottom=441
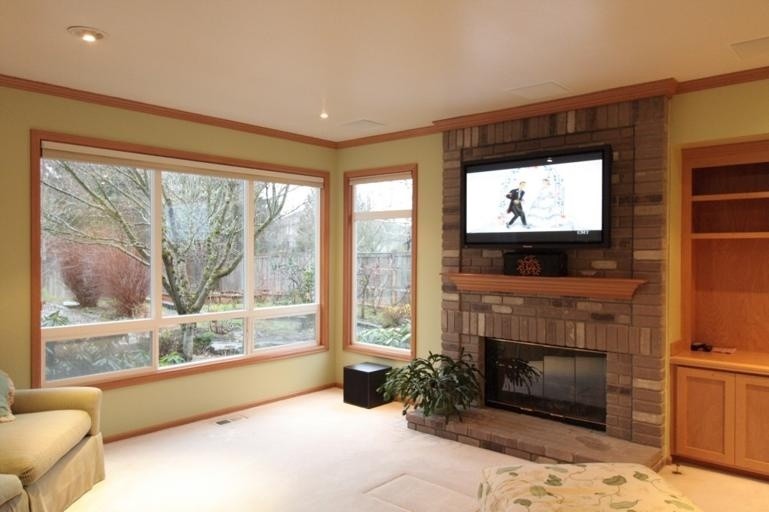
left=462, top=144, right=613, bottom=248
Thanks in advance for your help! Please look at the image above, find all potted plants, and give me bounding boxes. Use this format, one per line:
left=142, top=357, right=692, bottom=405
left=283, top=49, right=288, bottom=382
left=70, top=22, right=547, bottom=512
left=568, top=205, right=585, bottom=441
left=495, top=344, right=544, bottom=397
left=376, top=347, right=485, bottom=425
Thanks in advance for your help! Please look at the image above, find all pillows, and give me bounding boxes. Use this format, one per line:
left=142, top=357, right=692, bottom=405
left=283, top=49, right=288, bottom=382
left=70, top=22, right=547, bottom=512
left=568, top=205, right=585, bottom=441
left=0, top=369, right=16, bottom=423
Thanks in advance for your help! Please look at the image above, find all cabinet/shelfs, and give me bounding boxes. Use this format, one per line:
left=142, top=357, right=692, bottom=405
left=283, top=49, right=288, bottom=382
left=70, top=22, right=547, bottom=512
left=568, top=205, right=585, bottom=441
left=670, top=133, right=769, bottom=482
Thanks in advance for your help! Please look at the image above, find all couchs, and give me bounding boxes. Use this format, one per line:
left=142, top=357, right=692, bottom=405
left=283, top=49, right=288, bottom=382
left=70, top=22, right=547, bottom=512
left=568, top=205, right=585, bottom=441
left=0, top=386, right=105, bottom=512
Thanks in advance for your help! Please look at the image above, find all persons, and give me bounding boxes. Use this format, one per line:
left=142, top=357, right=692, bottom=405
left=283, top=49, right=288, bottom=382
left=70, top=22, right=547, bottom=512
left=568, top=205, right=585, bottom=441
left=503, top=180, right=529, bottom=227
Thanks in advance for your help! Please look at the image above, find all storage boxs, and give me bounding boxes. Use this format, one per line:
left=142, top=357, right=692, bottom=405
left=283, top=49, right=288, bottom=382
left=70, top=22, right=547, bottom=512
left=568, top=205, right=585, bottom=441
left=342, top=361, right=392, bottom=410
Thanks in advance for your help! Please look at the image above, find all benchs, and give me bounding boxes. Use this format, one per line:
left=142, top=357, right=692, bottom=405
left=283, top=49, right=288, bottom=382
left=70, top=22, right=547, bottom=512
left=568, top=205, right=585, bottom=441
left=473, top=461, right=703, bottom=511
